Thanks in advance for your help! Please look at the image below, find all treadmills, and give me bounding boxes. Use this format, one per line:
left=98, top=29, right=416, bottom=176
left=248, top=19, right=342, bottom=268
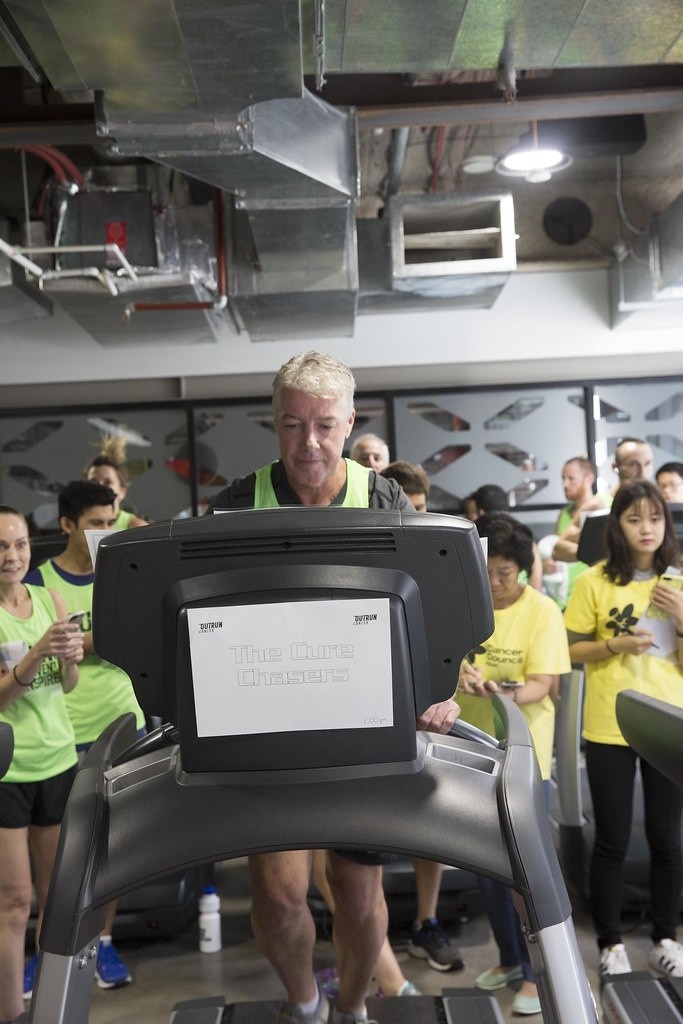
left=26, top=502, right=683, bottom=1023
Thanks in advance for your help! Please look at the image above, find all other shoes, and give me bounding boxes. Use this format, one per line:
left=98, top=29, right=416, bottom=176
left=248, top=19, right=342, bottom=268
left=476, top=964, right=523, bottom=990
left=373, top=979, right=422, bottom=997
left=277, top=980, right=329, bottom=1024
left=510, top=982, right=542, bottom=1014
left=313, top=965, right=342, bottom=999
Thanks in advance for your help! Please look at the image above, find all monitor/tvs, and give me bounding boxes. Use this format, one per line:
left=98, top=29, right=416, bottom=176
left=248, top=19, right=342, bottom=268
left=178, top=589, right=418, bottom=772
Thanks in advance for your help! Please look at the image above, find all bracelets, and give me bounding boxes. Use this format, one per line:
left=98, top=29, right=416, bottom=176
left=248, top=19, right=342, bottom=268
left=676, top=628, right=683, bottom=638
left=514, top=691, right=517, bottom=702
left=606, top=640, right=617, bottom=655
left=14, top=665, right=34, bottom=685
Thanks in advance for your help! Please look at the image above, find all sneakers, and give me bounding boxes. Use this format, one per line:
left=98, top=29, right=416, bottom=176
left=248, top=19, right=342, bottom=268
left=406, top=918, right=463, bottom=970
left=648, top=939, right=683, bottom=976
left=92, top=941, right=131, bottom=989
left=599, top=943, right=633, bottom=978
left=22, top=957, right=38, bottom=998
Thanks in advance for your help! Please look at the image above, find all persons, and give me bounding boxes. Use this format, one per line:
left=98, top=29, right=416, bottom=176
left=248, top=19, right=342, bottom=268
left=201, top=352, right=418, bottom=1024
left=0, top=433, right=683, bottom=1024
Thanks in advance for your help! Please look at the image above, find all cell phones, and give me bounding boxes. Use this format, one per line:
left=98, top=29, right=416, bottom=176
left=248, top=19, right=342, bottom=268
left=645, top=574, right=683, bottom=621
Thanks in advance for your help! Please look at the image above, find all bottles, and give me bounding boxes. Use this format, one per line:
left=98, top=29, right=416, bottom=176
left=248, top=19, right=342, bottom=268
left=198, top=886, right=223, bottom=953
